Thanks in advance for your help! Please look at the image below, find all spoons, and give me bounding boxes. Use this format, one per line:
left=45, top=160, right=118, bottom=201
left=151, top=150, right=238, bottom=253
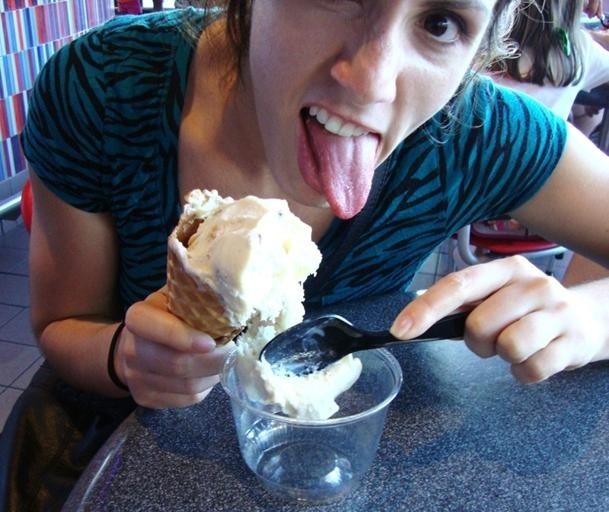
left=257, top=313, right=471, bottom=378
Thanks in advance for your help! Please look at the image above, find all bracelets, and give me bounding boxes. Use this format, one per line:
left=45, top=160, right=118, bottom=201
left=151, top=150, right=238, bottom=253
left=108, top=321, right=130, bottom=392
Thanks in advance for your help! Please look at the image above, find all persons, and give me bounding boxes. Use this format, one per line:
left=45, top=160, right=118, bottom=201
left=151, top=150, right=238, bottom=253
left=483, top=0, right=608, bottom=148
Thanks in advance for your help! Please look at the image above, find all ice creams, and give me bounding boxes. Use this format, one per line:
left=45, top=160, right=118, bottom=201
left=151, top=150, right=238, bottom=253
left=167, top=187, right=362, bottom=420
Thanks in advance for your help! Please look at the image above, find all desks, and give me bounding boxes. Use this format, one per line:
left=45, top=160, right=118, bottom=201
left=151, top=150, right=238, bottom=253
left=59, top=331, right=607, bottom=510
